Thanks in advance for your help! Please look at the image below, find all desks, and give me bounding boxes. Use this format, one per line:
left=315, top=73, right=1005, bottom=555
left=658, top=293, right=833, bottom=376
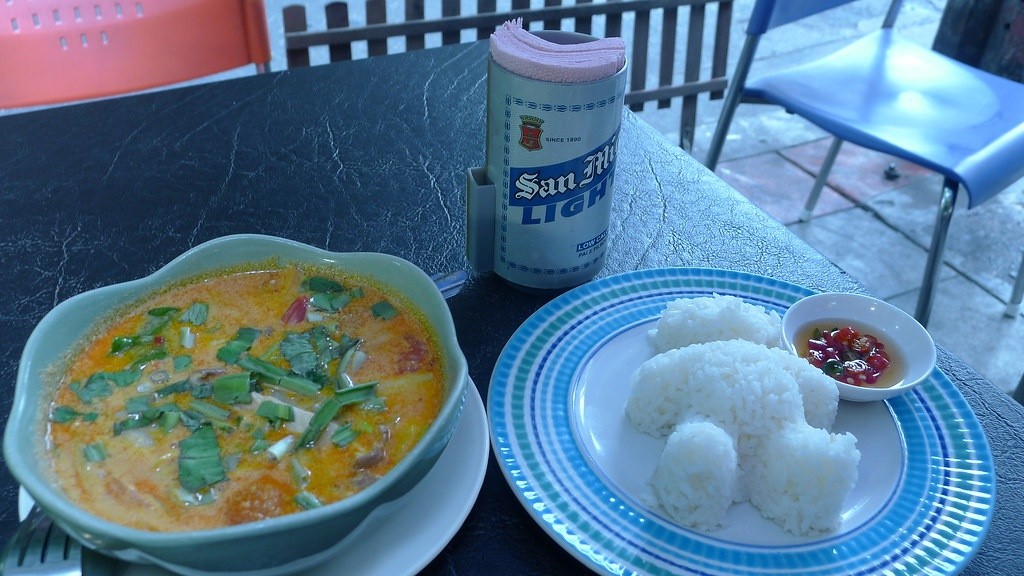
left=0, top=37, right=1024, bottom=576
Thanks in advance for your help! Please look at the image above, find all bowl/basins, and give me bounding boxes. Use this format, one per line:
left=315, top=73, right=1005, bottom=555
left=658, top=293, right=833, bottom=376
left=782, top=292, right=937, bottom=402
left=3, top=233, right=470, bottom=576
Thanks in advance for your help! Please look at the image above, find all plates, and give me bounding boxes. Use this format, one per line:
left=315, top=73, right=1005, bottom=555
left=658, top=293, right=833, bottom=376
left=488, top=266, right=996, bottom=576
left=18, top=376, right=490, bottom=576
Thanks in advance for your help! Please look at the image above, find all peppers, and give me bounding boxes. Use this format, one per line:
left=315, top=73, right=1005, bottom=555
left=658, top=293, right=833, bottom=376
left=809, top=325, right=890, bottom=384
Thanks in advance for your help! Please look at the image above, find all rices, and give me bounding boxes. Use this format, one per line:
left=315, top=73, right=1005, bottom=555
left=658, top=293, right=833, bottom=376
left=627, top=292, right=862, bottom=535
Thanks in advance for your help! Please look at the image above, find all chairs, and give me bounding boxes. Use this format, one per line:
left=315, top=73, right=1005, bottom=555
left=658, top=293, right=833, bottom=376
left=705, top=0, right=1024, bottom=329
left=0, top=0, right=271, bottom=110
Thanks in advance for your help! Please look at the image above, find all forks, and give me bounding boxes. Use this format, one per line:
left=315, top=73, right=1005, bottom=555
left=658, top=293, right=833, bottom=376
left=3, top=501, right=81, bottom=576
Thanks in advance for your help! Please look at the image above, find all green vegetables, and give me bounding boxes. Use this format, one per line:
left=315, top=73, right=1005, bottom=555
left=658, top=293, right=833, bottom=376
left=48, top=272, right=393, bottom=510
